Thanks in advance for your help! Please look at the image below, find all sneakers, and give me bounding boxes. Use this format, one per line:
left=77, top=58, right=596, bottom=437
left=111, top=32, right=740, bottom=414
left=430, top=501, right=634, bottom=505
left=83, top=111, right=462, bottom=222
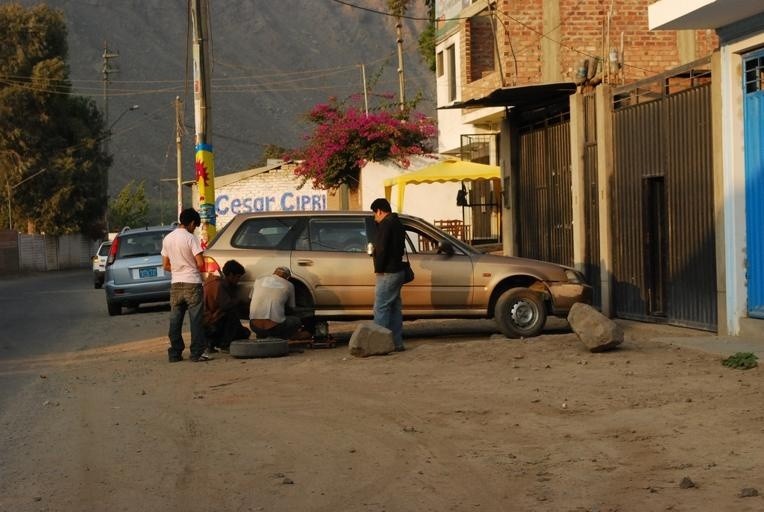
left=189, top=350, right=214, bottom=361
left=170, top=347, right=183, bottom=362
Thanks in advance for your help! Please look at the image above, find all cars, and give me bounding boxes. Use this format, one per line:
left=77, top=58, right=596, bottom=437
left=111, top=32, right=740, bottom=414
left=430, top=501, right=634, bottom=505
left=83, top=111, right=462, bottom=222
left=104, top=222, right=180, bottom=316
left=199, top=211, right=591, bottom=339
left=90, top=240, right=112, bottom=290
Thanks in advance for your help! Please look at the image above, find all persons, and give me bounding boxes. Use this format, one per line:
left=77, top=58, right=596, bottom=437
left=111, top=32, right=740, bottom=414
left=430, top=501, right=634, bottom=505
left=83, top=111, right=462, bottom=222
left=370, top=197, right=409, bottom=352
left=160, top=206, right=210, bottom=363
left=249, top=266, right=305, bottom=342
left=199, top=260, right=251, bottom=354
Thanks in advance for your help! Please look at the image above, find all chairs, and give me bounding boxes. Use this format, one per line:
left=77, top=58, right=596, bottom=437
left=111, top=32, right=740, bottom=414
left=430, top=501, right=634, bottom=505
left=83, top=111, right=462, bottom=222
left=417, top=219, right=471, bottom=252
left=244, top=231, right=271, bottom=249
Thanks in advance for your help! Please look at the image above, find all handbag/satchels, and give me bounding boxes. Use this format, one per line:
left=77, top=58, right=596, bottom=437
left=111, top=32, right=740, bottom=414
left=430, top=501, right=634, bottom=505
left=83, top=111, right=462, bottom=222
left=401, top=261, right=414, bottom=284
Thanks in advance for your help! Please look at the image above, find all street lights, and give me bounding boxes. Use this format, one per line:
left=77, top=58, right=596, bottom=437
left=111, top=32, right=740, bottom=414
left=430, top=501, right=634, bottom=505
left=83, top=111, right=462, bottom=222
left=95, top=103, right=140, bottom=234
